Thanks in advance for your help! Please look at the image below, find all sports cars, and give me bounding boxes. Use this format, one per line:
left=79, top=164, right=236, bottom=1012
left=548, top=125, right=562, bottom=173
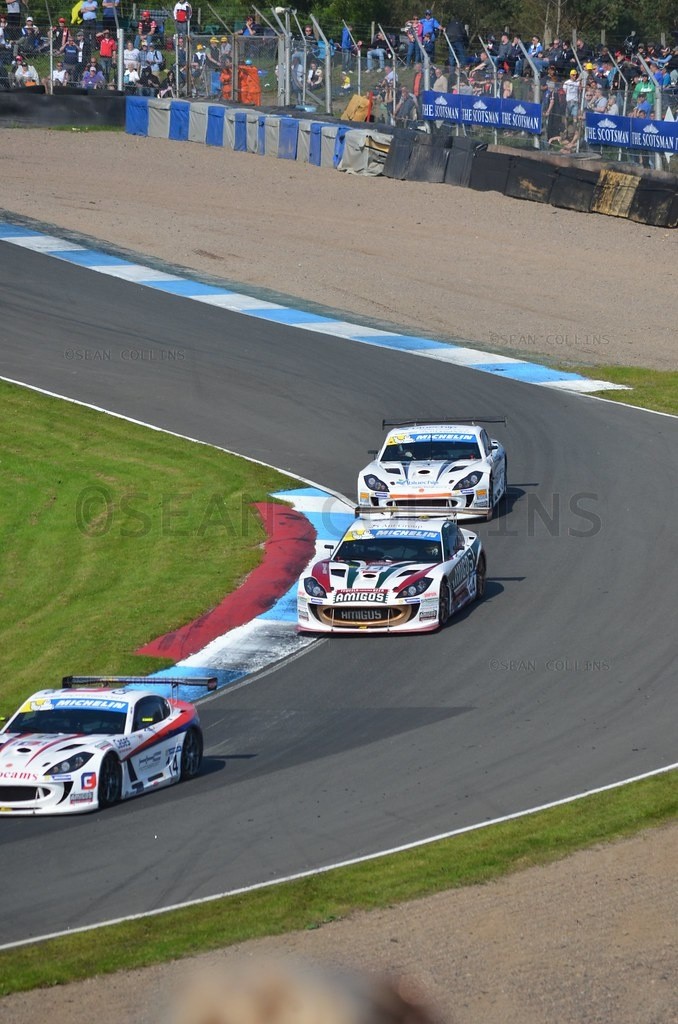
left=0, top=677, right=220, bottom=821
left=357, top=417, right=513, bottom=526
left=294, top=504, right=488, bottom=637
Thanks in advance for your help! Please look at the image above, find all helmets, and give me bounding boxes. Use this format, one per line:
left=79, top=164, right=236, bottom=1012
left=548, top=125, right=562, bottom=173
left=59, top=18, right=66, bottom=23
left=586, top=63, right=592, bottom=70
left=142, top=11, right=150, bottom=17
left=245, top=60, right=252, bottom=65
left=570, top=70, right=577, bottom=74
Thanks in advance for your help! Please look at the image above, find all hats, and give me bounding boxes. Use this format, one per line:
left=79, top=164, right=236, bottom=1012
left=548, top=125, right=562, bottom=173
left=647, top=41, right=655, bottom=47
left=21, top=62, right=28, bottom=66
left=141, top=41, right=148, bottom=47
left=149, top=42, right=155, bottom=48
left=637, top=92, right=647, bottom=97
left=90, top=67, right=96, bottom=71
left=16, top=56, right=22, bottom=60
left=485, top=74, right=491, bottom=78
left=672, top=46, right=678, bottom=51
left=196, top=44, right=203, bottom=50
left=210, top=37, right=219, bottom=42
left=26, top=16, right=33, bottom=21
left=220, top=37, right=227, bottom=42
left=641, top=71, right=649, bottom=76
left=638, top=43, right=645, bottom=48
left=497, top=69, right=506, bottom=74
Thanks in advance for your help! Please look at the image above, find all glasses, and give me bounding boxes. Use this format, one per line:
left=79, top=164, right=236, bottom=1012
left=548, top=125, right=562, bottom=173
left=57, top=65, right=63, bottom=67
left=586, top=94, right=592, bottom=96
left=90, top=61, right=96, bottom=63
left=639, top=47, right=643, bottom=49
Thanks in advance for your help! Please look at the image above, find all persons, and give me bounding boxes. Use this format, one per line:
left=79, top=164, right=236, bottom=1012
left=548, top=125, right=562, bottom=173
left=0, top=0, right=678, bottom=173
left=413, top=544, right=442, bottom=562
